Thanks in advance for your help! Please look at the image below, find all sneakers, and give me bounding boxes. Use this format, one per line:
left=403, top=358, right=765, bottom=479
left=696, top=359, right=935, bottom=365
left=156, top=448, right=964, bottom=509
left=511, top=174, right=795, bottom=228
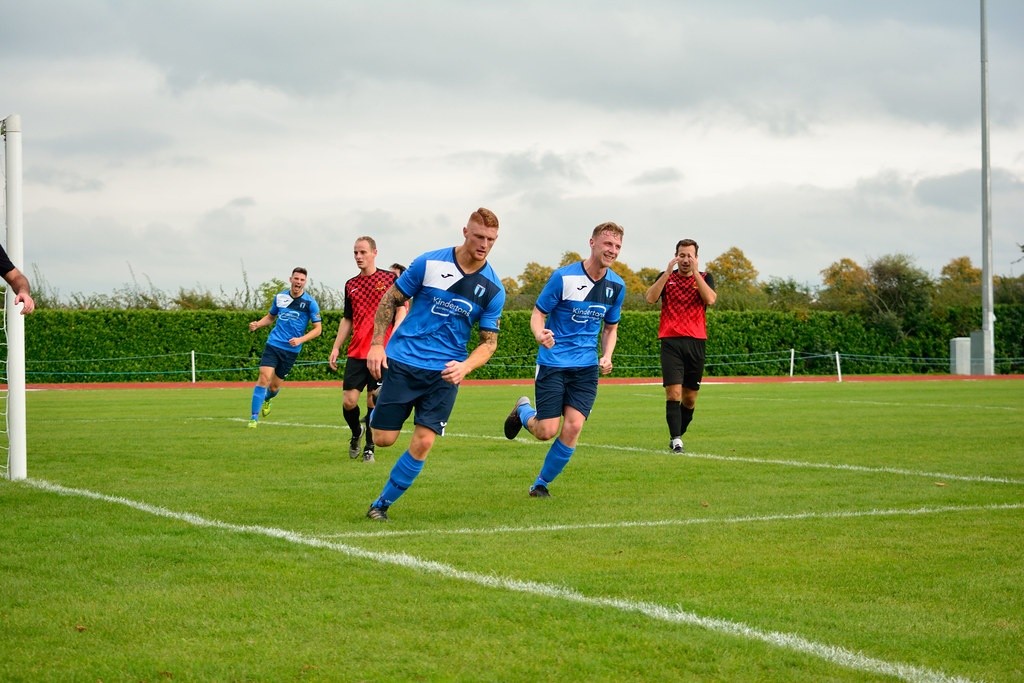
left=248, top=420, right=257, bottom=429
left=504, top=396, right=530, bottom=440
left=670, top=438, right=685, bottom=452
left=361, top=448, right=376, bottom=462
left=366, top=504, right=391, bottom=522
left=528, top=484, right=551, bottom=496
left=348, top=424, right=364, bottom=459
left=263, top=400, right=271, bottom=418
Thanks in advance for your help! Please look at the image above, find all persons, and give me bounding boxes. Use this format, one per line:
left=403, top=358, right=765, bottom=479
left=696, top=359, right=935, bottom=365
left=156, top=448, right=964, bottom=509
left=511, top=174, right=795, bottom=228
left=0, top=244, right=34, bottom=315
left=646, top=239, right=717, bottom=454
left=504, top=222, right=626, bottom=495
left=367, top=207, right=507, bottom=522
left=247, top=267, right=322, bottom=428
left=328, top=236, right=407, bottom=463
